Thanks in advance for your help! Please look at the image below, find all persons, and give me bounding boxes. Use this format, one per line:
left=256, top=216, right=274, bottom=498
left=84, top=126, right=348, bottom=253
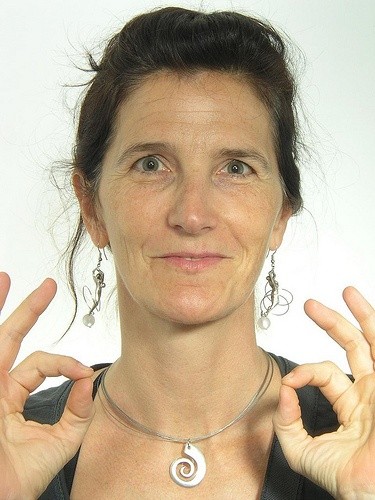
left=0, top=7, right=375, bottom=500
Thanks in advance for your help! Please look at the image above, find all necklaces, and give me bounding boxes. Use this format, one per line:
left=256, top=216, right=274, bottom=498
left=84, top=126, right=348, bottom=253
left=97, top=345, right=274, bottom=487
